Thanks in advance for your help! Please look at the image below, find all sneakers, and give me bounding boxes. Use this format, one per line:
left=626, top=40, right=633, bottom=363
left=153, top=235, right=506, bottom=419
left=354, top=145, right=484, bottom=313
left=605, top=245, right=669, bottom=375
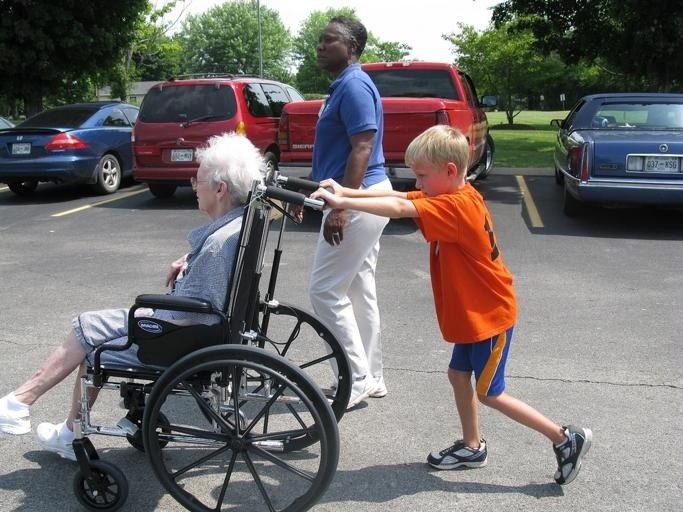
left=37, top=421, right=81, bottom=463
left=548, top=424, right=595, bottom=486
left=426, top=437, right=489, bottom=471
left=0, top=396, right=32, bottom=437
left=369, top=376, right=388, bottom=399
left=344, top=376, right=378, bottom=410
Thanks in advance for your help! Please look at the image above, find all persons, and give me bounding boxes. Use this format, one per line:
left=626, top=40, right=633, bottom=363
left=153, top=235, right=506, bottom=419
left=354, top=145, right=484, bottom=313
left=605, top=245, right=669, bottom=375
left=286, top=14, right=396, bottom=410
left=1, top=130, right=270, bottom=465
left=307, top=124, right=592, bottom=485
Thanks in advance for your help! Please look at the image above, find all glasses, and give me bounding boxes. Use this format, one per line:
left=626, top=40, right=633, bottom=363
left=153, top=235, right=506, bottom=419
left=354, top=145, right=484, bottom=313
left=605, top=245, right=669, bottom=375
left=189, top=176, right=211, bottom=192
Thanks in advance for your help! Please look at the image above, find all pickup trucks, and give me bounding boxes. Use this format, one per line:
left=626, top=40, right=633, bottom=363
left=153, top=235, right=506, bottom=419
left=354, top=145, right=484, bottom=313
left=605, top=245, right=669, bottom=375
left=272, top=55, right=496, bottom=221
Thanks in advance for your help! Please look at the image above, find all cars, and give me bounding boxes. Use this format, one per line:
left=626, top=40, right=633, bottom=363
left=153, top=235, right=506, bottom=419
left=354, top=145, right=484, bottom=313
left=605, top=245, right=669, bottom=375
left=0, top=100, right=143, bottom=197
left=0, top=113, right=16, bottom=131
left=549, top=92, right=682, bottom=214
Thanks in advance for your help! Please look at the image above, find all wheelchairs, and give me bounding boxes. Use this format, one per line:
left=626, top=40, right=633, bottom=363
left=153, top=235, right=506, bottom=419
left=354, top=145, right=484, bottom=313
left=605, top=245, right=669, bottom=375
left=68, top=177, right=349, bottom=509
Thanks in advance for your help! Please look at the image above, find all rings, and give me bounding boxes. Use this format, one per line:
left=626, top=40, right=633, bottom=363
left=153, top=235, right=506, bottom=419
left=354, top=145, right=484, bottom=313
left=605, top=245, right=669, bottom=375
left=331, top=231, right=338, bottom=236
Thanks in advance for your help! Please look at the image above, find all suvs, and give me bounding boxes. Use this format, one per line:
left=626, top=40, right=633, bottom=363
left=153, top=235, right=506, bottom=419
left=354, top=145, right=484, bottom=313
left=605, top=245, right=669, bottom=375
left=126, top=69, right=305, bottom=198
